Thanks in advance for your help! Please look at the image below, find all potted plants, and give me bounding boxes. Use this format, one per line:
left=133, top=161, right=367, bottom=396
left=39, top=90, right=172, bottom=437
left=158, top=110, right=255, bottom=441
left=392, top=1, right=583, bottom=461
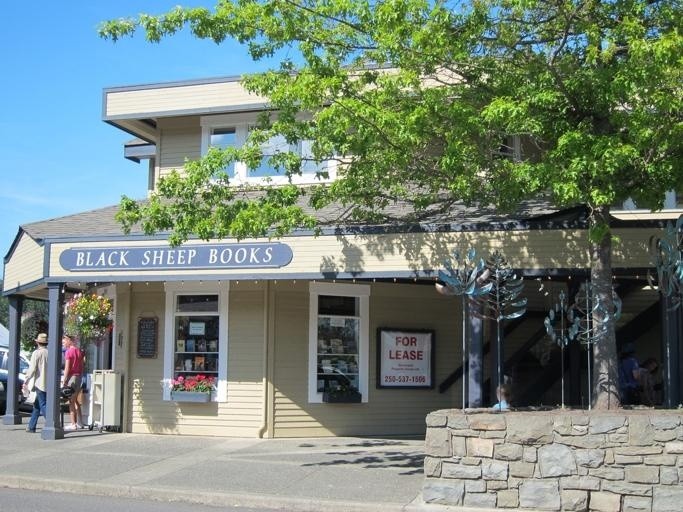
left=323, top=385, right=362, bottom=403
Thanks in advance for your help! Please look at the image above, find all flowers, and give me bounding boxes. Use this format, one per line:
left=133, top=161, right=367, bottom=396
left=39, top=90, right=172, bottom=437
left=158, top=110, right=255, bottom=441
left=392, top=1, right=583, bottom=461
left=60, top=290, right=114, bottom=336
left=171, top=375, right=217, bottom=394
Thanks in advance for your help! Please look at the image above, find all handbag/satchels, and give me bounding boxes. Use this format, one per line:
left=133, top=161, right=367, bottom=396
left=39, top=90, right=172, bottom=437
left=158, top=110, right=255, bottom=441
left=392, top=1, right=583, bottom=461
left=60, top=385, right=75, bottom=397
left=22, top=391, right=37, bottom=405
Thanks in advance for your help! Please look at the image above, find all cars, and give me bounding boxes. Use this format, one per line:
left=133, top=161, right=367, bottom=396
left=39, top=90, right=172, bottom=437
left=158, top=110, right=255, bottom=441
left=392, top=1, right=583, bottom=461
left=0, top=345, right=71, bottom=413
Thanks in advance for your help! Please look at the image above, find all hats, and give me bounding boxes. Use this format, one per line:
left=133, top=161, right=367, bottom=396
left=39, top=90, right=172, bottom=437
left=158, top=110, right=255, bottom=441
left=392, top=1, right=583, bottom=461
left=34, top=333, right=48, bottom=344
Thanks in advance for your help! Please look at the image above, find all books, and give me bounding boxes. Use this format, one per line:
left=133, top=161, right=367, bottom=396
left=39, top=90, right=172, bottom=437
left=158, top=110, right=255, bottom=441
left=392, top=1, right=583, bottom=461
left=177, top=339, right=219, bottom=371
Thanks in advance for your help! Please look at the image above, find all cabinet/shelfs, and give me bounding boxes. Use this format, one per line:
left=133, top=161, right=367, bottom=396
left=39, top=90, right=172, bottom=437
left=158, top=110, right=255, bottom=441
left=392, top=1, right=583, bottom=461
left=316, top=314, right=360, bottom=393
left=173, top=312, right=219, bottom=372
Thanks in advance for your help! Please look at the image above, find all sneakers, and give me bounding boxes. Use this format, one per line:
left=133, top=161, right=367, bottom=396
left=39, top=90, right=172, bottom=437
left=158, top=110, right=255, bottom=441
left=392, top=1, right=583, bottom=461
left=64, top=422, right=83, bottom=429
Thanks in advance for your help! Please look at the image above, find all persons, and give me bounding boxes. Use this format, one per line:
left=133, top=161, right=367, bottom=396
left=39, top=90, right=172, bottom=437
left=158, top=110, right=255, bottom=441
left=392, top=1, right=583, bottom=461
left=22, top=333, right=86, bottom=433
left=491, top=383, right=516, bottom=409
left=618, top=343, right=660, bottom=405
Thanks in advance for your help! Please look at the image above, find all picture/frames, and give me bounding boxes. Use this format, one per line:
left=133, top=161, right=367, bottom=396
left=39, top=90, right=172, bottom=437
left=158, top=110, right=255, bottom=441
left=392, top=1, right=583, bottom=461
left=375, top=327, right=435, bottom=389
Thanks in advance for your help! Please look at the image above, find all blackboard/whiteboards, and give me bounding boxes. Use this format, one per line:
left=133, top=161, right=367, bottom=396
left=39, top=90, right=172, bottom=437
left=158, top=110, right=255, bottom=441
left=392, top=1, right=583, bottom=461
left=136, top=317, right=158, bottom=358
